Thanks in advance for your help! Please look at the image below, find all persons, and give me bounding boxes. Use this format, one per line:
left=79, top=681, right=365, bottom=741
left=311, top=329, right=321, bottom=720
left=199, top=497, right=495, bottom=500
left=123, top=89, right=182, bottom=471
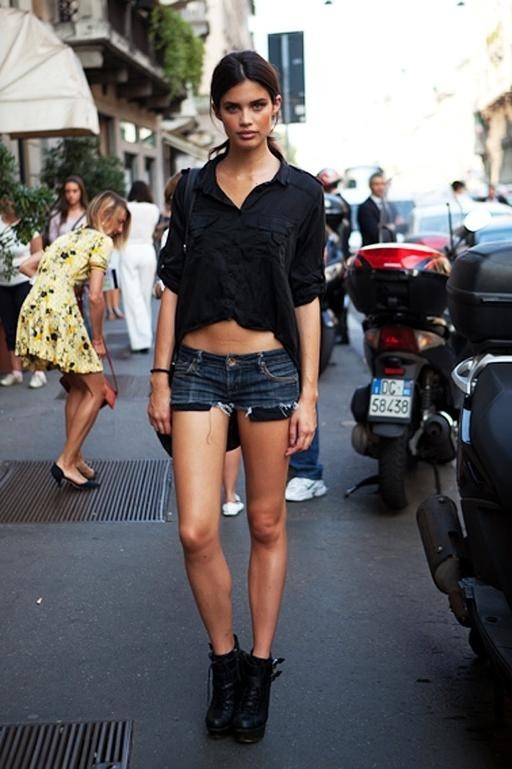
left=222, top=402, right=327, bottom=515
left=148, top=49, right=326, bottom=744
left=319, top=171, right=511, bottom=345
left=1, top=176, right=183, bottom=490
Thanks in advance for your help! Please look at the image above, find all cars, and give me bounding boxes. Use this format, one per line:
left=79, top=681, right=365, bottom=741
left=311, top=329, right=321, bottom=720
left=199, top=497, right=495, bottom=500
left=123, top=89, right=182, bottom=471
left=326, top=166, right=510, bottom=253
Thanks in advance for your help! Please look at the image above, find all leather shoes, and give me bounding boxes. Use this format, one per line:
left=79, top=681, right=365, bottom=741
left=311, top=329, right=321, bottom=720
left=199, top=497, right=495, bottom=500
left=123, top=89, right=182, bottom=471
left=205, top=634, right=247, bottom=739
left=234, top=647, right=284, bottom=743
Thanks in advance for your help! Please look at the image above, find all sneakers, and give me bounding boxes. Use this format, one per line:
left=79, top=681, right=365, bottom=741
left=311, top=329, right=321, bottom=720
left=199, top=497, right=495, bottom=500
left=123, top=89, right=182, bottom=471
left=286, top=474, right=328, bottom=501
left=1, top=373, right=24, bottom=386
left=222, top=497, right=243, bottom=516
left=30, top=371, right=48, bottom=388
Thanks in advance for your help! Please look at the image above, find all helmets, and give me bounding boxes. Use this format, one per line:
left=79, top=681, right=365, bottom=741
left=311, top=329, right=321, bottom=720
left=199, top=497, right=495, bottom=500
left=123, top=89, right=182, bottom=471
left=319, top=168, right=342, bottom=190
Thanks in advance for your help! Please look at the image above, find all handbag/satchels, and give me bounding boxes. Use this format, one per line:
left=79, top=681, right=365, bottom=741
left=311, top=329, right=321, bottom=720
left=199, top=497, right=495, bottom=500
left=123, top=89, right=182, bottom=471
left=144, top=387, right=239, bottom=461
left=59, top=366, right=117, bottom=411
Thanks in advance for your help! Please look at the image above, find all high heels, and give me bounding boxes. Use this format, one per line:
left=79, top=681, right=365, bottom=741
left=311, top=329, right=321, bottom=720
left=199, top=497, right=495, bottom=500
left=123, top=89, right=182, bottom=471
left=51, top=461, right=99, bottom=489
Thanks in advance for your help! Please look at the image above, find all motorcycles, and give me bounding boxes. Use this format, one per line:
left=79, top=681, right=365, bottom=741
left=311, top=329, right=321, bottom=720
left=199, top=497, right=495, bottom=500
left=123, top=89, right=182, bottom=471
left=318, top=194, right=353, bottom=379
left=412, top=239, right=511, bottom=676
left=342, top=208, right=493, bottom=513
